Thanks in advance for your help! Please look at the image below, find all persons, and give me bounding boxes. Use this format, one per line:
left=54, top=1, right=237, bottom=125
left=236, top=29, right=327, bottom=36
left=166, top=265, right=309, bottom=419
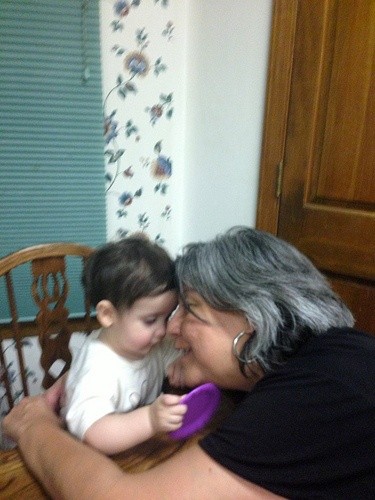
left=57, top=234, right=189, bottom=457
left=1, top=224, right=375, bottom=500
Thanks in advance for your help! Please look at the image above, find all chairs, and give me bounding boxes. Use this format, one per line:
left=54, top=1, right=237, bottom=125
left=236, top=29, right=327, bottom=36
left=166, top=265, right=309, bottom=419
left=0, top=241, right=97, bottom=438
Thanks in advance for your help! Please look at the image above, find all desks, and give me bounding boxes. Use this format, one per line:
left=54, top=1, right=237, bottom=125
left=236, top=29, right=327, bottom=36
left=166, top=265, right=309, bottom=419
left=0, top=412, right=226, bottom=500
